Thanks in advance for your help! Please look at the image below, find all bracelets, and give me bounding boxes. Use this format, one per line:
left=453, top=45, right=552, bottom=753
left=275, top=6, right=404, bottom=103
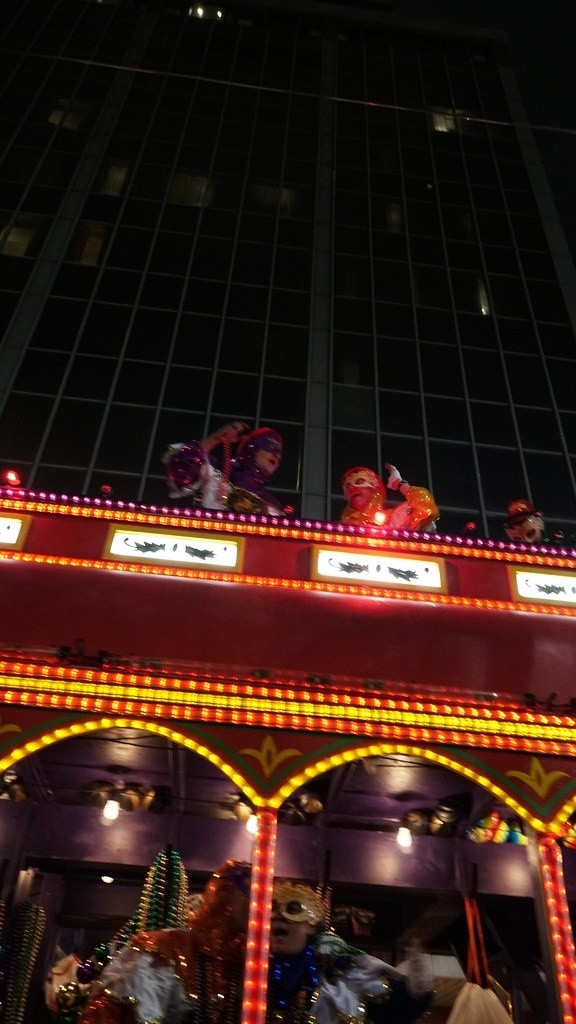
left=398, top=480, right=408, bottom=492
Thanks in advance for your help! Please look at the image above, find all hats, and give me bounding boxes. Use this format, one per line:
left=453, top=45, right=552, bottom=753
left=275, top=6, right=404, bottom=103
left=236, top=427, right=284, bottom=461
left=506, top=499, right=536, bottom=528
left=341, top=466, right=387, bottom=503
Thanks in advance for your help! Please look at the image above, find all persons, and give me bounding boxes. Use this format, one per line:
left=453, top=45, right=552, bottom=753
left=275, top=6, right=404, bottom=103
left=161, top=420, right=296, bottom=519
left=502, top=498, right=576, bottom=549
left=77, top=859, right=253, bottom=1024
left=265, top=879, right=437, bottom=1024
left=338, top=463, right=441, bottom=533
